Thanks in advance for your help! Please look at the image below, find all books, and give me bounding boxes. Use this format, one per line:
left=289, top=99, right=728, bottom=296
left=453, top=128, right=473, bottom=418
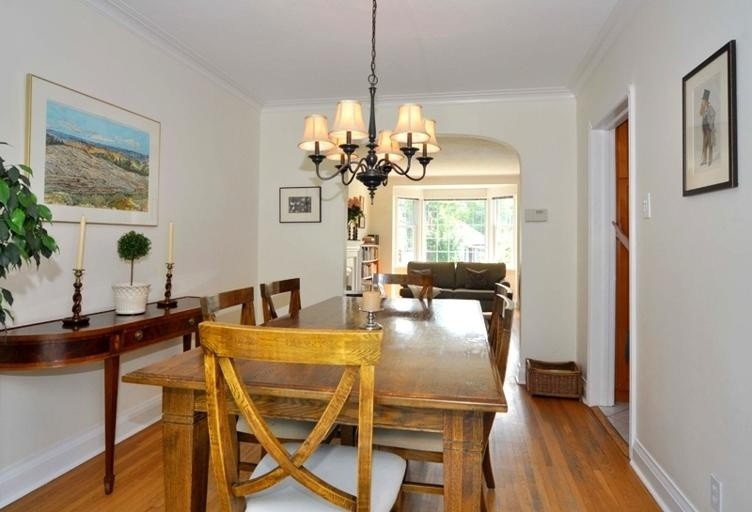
left=362, top=246, right=378, bottom=280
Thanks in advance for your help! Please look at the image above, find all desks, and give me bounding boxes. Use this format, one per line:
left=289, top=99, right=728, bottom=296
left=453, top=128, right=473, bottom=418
left=0, top=295, right=208, bottom=495
left=121, top=295, right=512, bottom=512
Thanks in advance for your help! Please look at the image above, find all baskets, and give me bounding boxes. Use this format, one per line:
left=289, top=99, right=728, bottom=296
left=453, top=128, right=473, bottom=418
left=523, top=353, right=583, bottom=400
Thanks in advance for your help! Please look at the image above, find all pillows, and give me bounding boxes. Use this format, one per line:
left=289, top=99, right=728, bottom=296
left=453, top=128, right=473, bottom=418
left=464, top=267, right=490, bottom=290
left=408, top=268, right=431, bottom=285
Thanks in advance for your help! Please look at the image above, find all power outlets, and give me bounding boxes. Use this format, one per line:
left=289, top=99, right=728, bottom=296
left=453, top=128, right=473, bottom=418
left=708, top=472, right=723, bottom=512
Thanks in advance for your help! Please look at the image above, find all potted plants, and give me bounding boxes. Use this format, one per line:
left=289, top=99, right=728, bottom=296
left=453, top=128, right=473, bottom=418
left=111, top=230, right=152, bottom=316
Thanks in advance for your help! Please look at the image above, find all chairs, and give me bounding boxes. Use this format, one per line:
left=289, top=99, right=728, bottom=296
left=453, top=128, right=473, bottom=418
left=369, top=271, right=434, bottom=301
left=488, top=283, right=513, bottom=352
left=260, top=276, right=303, bottom=326
left=197, top=320, right=408, bottom=512
left=360, top=293, right=513, bottom=512
left=197, top=287, right=325, bottom=476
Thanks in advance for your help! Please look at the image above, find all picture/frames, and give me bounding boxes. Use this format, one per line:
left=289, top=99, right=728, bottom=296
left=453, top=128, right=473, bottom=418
left=23, top=73, right=163, bottom=229
left=679, top=37, right=740, bottom=197
left=279, top=185, right=322, bottom=224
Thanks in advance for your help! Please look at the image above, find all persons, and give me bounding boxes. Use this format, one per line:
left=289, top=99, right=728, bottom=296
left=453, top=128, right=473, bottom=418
left=696, top=88, right=716, bottom=168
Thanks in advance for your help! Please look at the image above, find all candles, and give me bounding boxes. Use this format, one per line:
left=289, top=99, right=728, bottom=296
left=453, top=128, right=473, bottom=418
left=167, top=222, right=175, bottom=265
left=77, top=215, right=87, bottom=269
left=361, top=289, right=384, bottom=310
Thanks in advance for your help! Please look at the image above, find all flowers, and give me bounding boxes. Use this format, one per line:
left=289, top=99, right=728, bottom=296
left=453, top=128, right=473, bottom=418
left=347, top=196, right=366, bottom=226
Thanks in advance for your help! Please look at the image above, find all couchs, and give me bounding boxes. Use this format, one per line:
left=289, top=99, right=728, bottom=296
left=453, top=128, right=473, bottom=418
left=405, top=261, right=512, bottom=313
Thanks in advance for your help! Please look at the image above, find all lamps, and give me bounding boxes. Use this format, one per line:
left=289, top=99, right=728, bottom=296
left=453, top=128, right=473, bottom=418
left=297, top=1, right=443, bottom=208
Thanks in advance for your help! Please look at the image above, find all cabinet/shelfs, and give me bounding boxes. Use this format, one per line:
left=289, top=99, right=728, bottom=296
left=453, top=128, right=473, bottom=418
left=360, top=243, right=379, bottom=284
left=347, top=239, right=364, bottom=295
